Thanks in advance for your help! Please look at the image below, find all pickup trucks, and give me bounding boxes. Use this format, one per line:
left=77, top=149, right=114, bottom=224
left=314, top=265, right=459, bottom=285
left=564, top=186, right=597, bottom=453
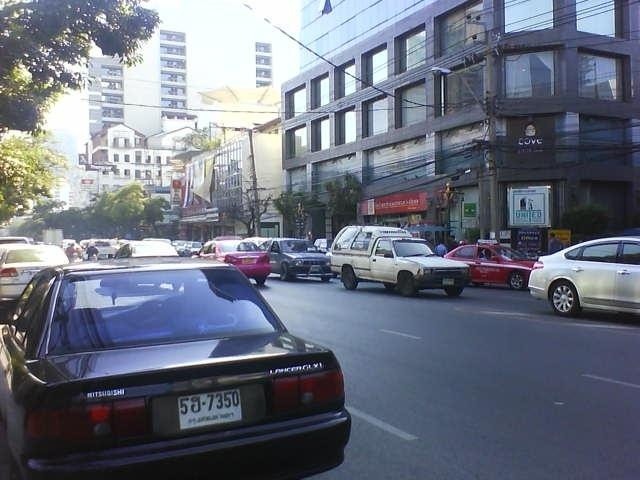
left=328, top=226, right=472, bottom=300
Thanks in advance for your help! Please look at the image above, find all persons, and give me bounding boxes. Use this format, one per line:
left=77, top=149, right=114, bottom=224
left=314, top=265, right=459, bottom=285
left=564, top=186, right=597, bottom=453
left=434, top=239, right=448, bottom=258
left=446, top=235, right=459, bottom=251
left=64, top=242, right=77, bottom=262
left=547, top=233, right=563, bottom=255
left=84, top=242, right=99, bottom=262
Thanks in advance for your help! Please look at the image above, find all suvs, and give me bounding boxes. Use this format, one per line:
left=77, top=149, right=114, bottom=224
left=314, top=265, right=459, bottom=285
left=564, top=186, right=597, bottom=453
left=0, top=236, right=31, bottom=254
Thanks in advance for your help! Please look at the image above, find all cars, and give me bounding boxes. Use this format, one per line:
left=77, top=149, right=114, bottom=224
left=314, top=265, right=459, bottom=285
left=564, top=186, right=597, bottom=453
left=0, top=254, right=352, bottom=480
left=0, top=245, right=70, bottom=311
left=443, top=244, right=536, bottom=292
left=528, top=236, right=640, bottom=318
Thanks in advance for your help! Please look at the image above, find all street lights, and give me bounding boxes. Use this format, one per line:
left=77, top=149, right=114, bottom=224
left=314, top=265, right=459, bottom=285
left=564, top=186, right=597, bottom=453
left=430, top=66, right=500, bottom=239
